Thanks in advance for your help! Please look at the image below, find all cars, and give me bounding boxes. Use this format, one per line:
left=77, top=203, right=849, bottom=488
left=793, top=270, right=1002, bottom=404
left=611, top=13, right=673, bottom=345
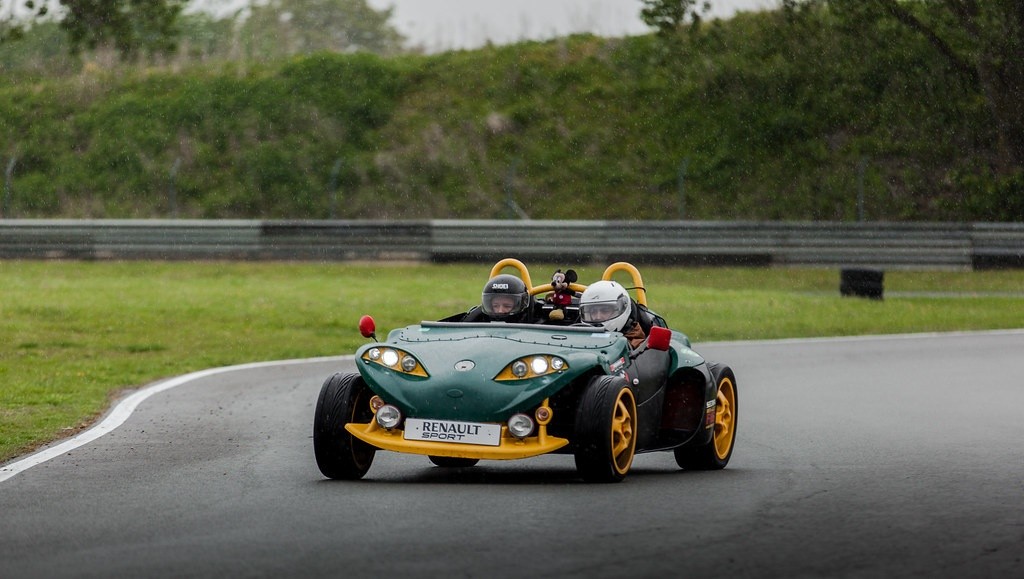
left=313, top=258, right=738, bottom=481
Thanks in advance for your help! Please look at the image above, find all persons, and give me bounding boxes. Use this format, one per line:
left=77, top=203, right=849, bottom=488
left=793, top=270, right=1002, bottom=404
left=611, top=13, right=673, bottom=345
left=579, top=281, right=646, bottom=351
left=482, top=273, right=531, bottom=323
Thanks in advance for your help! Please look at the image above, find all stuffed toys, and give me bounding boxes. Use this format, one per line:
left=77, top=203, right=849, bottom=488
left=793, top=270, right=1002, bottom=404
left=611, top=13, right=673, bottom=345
left=548, top=269, right=577, bottom=322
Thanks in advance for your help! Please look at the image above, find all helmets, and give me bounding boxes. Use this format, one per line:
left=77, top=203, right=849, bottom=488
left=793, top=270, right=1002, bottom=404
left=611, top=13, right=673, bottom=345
left=482, top=274, right=529, bottom=315
left=579, top=280, right=631, bottom=331
